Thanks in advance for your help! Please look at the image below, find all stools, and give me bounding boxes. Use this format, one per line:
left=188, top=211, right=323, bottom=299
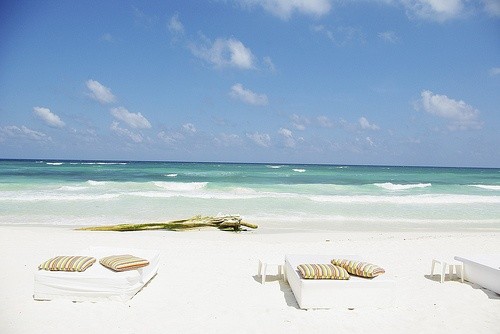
left=431, top=257, right=464, bottom=284
left=257, top=258, right=285, bottom=286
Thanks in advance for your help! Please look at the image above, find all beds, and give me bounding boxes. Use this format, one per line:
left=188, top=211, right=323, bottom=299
left=34, top=243, right=159, bottom=303
left=454, top=251, right=500, bottom=295
left=283, top=251, right=388, bottom=308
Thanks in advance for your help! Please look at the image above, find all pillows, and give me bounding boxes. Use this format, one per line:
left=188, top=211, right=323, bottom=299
left=331, top=257, right=387, bottom=278
left=297, top=263, right=349, bottom=280
left=99, top=253, right=150, bottom=271
left=37, top=254, right=97, bottom=272
